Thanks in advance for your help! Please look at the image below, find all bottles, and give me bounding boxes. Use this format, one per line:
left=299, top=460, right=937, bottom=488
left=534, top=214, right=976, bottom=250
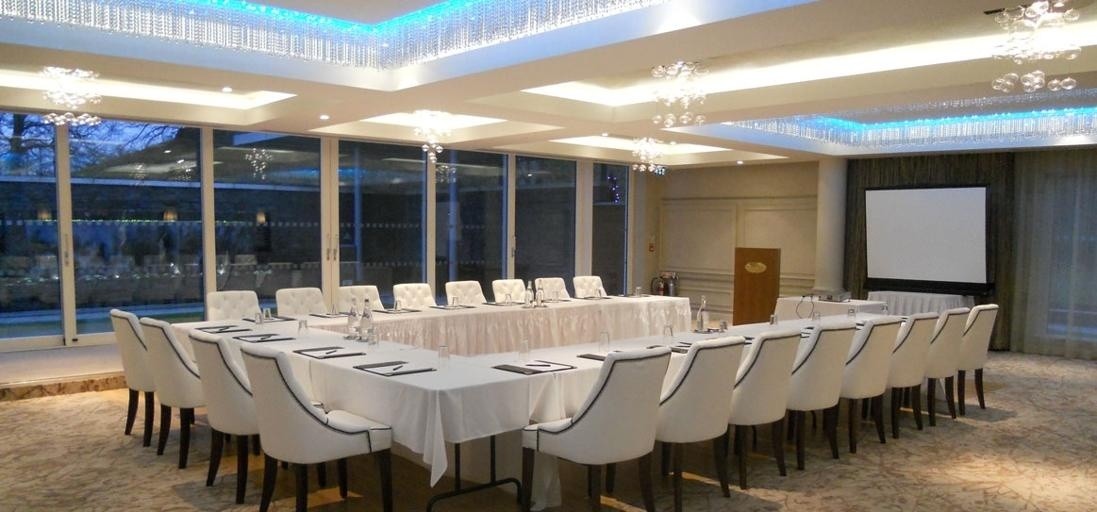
left=346, top=279, right=709, bottom=337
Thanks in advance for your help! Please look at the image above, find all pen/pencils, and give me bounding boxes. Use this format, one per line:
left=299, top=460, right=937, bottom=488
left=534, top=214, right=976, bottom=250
left=526, top=363, right=552, bottom=368
left=393, top=365, right=403, bottom=370
left=325, top=350, right=337, bottom=354
left=261, top=336, right=271, bottom=339
left=219, top=326, right=229, bottom=331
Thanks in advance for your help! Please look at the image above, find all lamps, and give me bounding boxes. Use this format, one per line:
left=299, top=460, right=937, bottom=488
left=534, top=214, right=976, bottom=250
left=245, top=147, right=273, bottom=180
left=39, top=66, right=103, bottom=127
left=436, top=164, right=458, bottom=185
left=632, top=136, right=677, bottom=176
left=991, top=1, right=1081, bottom=92
left=410, top=110, right=452, bottom=164
left=650, top=61, right=712, bottom=127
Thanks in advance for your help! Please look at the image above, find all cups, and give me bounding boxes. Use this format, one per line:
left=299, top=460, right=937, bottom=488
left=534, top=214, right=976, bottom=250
left=254, top=286, right=856, bottom=364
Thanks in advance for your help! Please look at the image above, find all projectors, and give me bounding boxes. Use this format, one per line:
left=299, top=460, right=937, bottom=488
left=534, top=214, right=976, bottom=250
left=819, top=289, right=852, bottom=303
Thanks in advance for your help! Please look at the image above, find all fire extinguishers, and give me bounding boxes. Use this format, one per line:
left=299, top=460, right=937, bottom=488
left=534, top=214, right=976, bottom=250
left=664, top=272, right=678, bottom=296
left=650, top=275, right=666, bottom=296
